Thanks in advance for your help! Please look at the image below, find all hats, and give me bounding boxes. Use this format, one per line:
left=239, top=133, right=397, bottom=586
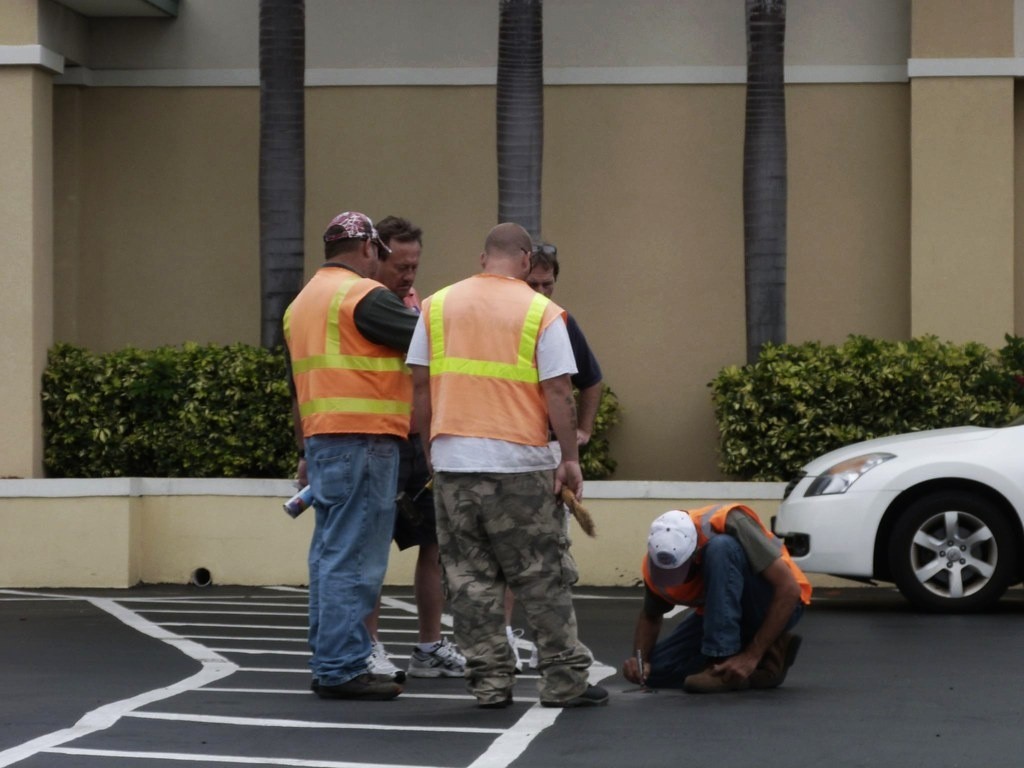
left=324, top=211, right=393, bottom=255
left=647, top=509, right=699, bottom=587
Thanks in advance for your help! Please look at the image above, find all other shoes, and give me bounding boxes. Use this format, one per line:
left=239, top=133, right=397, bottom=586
left=540, top=683, right=610, bottom=707
left=483, top=691, right=513, bottom=709
left=311, top=679, right=319, bottom=694
left=317, top=674, right=402, bottom=699
left=751, top=633, right=802, bottom=690
left=682, top=664, right=753, bottom=692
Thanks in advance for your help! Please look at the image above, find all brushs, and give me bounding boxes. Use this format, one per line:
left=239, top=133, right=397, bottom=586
left=560, top=484, right=597, bottom=541
left=636, top=648, right=649, bottom=692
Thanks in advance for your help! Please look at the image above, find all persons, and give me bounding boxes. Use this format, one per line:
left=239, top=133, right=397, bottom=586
left=406, top=222, right=608, bottom=709
left=281, top=212, right=420, bottom=699
left=366, top=217, right=466, bottom=678
left=622, top=503, right=812, bottom=692
left=506, top=245, right=602, bottom=672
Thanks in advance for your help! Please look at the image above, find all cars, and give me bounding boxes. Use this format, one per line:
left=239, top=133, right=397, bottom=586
left=770, top=416, right=1023, bottom=616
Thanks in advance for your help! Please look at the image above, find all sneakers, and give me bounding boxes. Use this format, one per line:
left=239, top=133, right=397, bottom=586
left=528, top=644, right=538, bottom=669
left=366, top=635, right=406, bottom=682
left=408, top=637, right=470, bottom=677
left=507, top=629, right=525, bottom=673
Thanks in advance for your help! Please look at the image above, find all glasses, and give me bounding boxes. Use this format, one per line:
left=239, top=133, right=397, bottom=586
left=531, top=244, right=557, bottom=255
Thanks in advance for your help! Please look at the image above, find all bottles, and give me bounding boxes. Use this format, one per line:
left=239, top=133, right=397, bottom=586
left=284, top=484, right=313, bottom=518
